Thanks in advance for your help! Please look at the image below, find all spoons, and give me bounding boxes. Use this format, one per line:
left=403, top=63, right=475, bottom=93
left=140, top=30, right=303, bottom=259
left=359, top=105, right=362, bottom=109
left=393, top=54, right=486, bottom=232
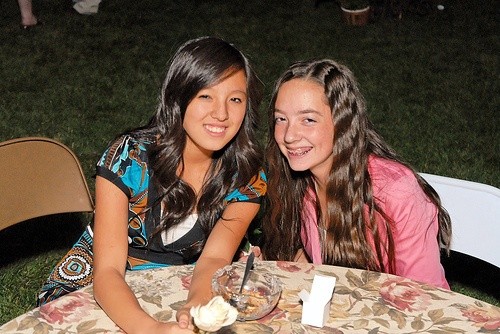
left=228, top=251, right=254, bottom=312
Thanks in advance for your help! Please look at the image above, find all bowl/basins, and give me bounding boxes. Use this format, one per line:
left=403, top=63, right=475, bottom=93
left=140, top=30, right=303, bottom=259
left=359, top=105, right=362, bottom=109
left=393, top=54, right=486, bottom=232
left=209, top=262, right=282, bottom=322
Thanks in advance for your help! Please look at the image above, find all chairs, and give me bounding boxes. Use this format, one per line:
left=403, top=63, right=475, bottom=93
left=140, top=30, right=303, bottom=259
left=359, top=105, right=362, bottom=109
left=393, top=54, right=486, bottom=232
left=0, top=137, right=95, bottom=231
left=417, top=172, right=500, bottom=267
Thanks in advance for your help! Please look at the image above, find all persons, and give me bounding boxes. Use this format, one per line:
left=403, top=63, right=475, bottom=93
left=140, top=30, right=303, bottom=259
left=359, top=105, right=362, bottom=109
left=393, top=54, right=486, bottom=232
left=239, top=56, right=453, bottom=292
left=16, top=0, right=38, bottom=26
left=35, top=34, right=268, bottom=334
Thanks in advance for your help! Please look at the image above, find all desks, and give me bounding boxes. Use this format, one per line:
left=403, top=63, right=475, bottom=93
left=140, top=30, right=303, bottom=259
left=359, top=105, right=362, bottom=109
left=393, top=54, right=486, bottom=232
left=0, top=260, right=500, bottom=334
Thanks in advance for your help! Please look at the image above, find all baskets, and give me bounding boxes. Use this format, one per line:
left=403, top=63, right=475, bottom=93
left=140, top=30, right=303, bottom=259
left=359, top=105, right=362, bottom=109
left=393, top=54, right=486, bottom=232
left=340, top=6, right=370, bottom=28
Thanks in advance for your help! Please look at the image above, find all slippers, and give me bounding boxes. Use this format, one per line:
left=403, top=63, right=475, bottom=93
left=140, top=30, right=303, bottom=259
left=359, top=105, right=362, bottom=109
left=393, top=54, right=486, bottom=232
left=20, top=17, right=44, bottom=30
